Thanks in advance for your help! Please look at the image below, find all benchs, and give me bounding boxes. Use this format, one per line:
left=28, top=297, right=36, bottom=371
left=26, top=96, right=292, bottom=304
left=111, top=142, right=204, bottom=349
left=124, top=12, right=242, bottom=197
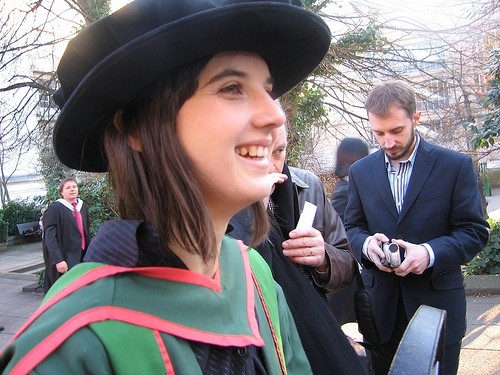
left=16, top=221, right=42, bottom=244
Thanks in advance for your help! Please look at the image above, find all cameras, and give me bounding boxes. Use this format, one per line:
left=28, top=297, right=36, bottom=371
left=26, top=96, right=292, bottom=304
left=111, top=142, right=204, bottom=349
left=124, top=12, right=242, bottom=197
left=382, top=243, right=401, bottom=267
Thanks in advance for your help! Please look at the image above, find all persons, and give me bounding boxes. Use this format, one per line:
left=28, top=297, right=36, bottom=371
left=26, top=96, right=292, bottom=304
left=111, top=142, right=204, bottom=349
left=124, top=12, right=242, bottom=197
left=344, top=79, right=490, bottom=375
left=331, top=137, right=370, bottom=228
left=0, top=0, right=331, bottom=375
left=42, top=176, right=91, bottom=295
left=229, top=98, right=356, bottom=375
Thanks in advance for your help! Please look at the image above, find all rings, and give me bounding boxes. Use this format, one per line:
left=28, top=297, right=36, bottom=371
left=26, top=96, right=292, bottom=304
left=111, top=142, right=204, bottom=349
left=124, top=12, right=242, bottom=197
left=309, top=247, right=312, bottom=256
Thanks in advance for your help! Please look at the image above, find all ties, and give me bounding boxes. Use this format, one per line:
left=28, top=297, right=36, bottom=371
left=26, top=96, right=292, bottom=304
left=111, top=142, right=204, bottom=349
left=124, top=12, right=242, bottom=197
left=72, top=202, right=86, bottom=250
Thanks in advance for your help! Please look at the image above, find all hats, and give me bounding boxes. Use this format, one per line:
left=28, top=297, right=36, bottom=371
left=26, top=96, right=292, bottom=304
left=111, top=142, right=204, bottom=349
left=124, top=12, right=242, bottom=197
left=53, top=0, right=331, bottom=172
